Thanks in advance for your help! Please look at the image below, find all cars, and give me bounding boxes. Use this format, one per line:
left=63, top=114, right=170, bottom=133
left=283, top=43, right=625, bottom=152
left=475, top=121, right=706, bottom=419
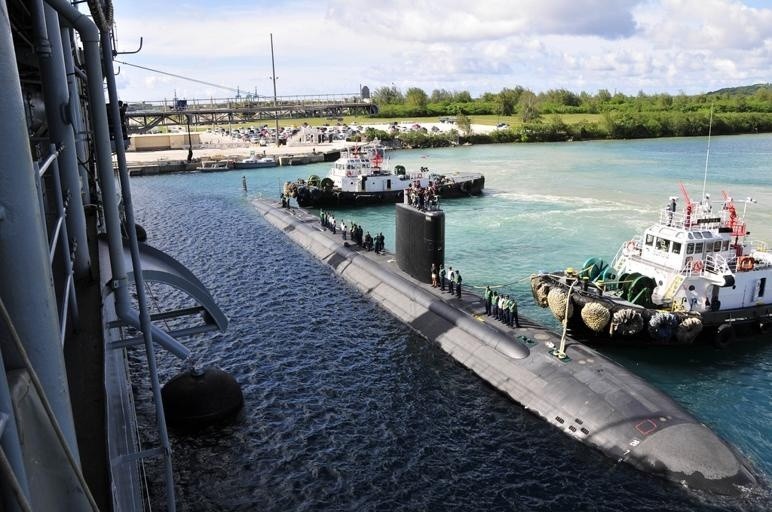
left=387, top=122, right=443, bottom=136
left=202, top=121, right=364, bottom=147
left=497, top=122, right=509, bottom=129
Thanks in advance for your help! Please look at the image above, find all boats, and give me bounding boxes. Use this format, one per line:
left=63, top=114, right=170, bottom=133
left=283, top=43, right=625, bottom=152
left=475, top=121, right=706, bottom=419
left=284, top=142, right=486, bottom=207
left=528, top=101, right=772, bottom=341
left=193, top=147, right=278, bottom=172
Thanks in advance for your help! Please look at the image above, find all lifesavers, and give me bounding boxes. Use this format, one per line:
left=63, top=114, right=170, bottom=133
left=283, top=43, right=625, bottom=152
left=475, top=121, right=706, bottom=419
left=346, top=171, right=352, bottom=177
left=739, top=257, right=754, bottom=272
left=627, top=241, right=635, bottom=250
left=418, top=173, right=422, bottom=178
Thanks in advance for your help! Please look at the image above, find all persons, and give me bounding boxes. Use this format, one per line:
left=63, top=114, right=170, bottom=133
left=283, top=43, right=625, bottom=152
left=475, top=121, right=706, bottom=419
left=408, top=180, right=440, bottom=211
left=447, top=267, right=454, bottom=295
left=439, top=265, right=446, bottom=291
left=483, top=286, right=519, bottom=327
left=431, top=263, right=438, bottom=288
left=451, top=270, right=462, bottom=298
left=281, top=193, right=290, bottom=209
left=220, top=122, right=400, bottom=146
left=319, top=208, right=384, bottom=254
